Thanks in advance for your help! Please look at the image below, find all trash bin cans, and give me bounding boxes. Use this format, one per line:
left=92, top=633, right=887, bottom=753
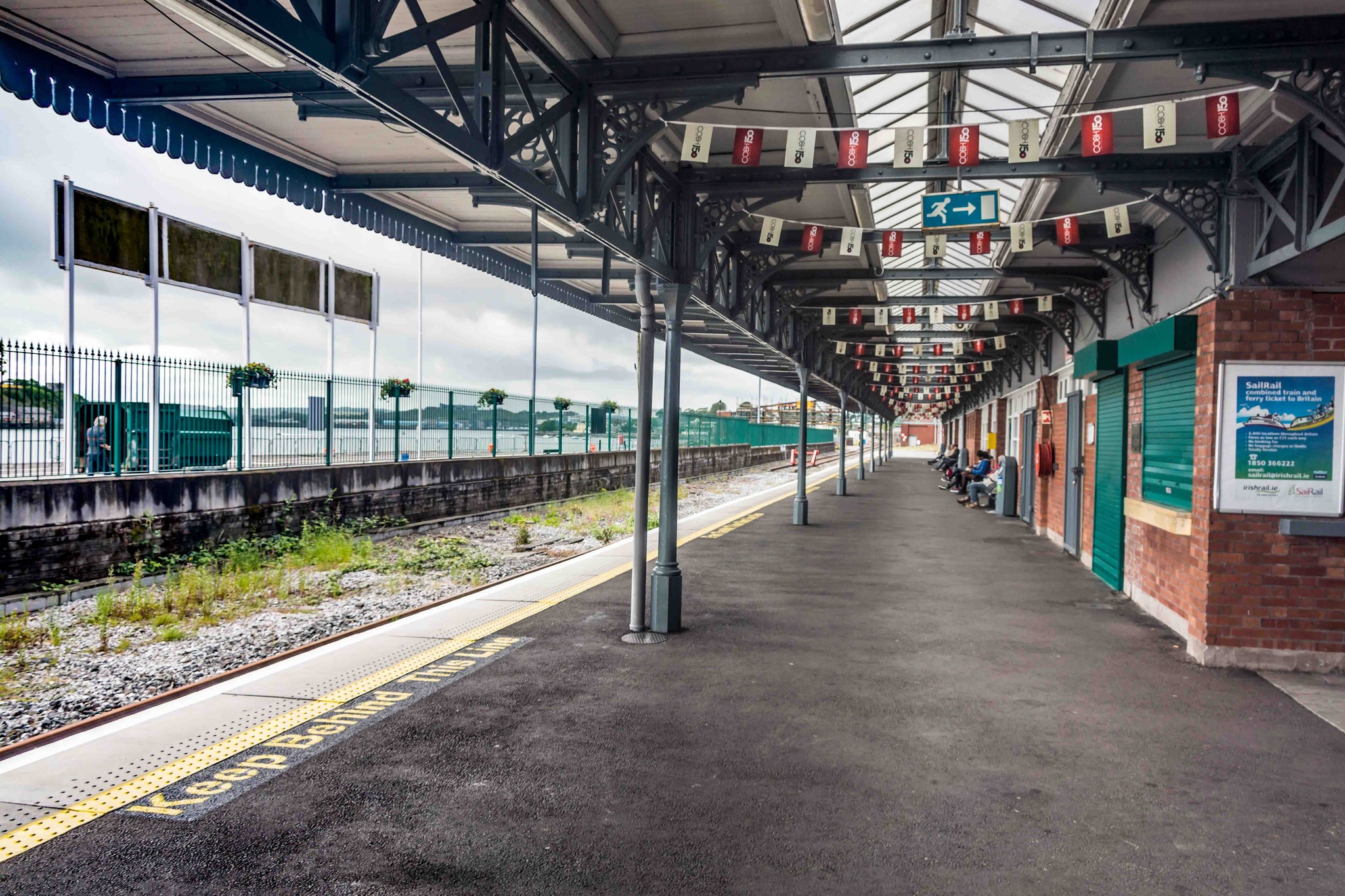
left=995, top=454, right=1017, bottom=516
left=956, top=448, right=968, bottom=470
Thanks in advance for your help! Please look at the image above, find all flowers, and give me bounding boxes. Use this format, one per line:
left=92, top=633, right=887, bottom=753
left=225, top=362, right=281, bottom=389
left=378, top=378, right=418, bottom=403
left=477, top=389, right=508, bottom=408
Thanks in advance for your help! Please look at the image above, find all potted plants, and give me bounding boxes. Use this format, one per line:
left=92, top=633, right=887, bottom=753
left=600, top=400, right=620, bottom=413
left=554, top=397, right=573, bottom=410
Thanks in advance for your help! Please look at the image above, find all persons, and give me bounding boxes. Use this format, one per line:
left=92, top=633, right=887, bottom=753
left=86, top=415, right=111, bottom=475
left=966, top=454, right=1003, bottom=509
left=927, top=444, right=991, bottom=495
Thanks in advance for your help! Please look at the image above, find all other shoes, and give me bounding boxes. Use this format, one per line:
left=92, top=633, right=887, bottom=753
left=965, top=501, right=979, bottom=509
left=928, top=461, right=946, bottom=476
left=957, top=496, right=969, bottom=503
left=938, top=476, right=966, bottom=495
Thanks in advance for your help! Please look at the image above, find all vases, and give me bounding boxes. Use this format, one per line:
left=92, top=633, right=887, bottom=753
left=388, top=387, right=410, bottom=397
left=244, top=371, right=272, bottom=388
left=488, top=397, right=503, bottom=405
